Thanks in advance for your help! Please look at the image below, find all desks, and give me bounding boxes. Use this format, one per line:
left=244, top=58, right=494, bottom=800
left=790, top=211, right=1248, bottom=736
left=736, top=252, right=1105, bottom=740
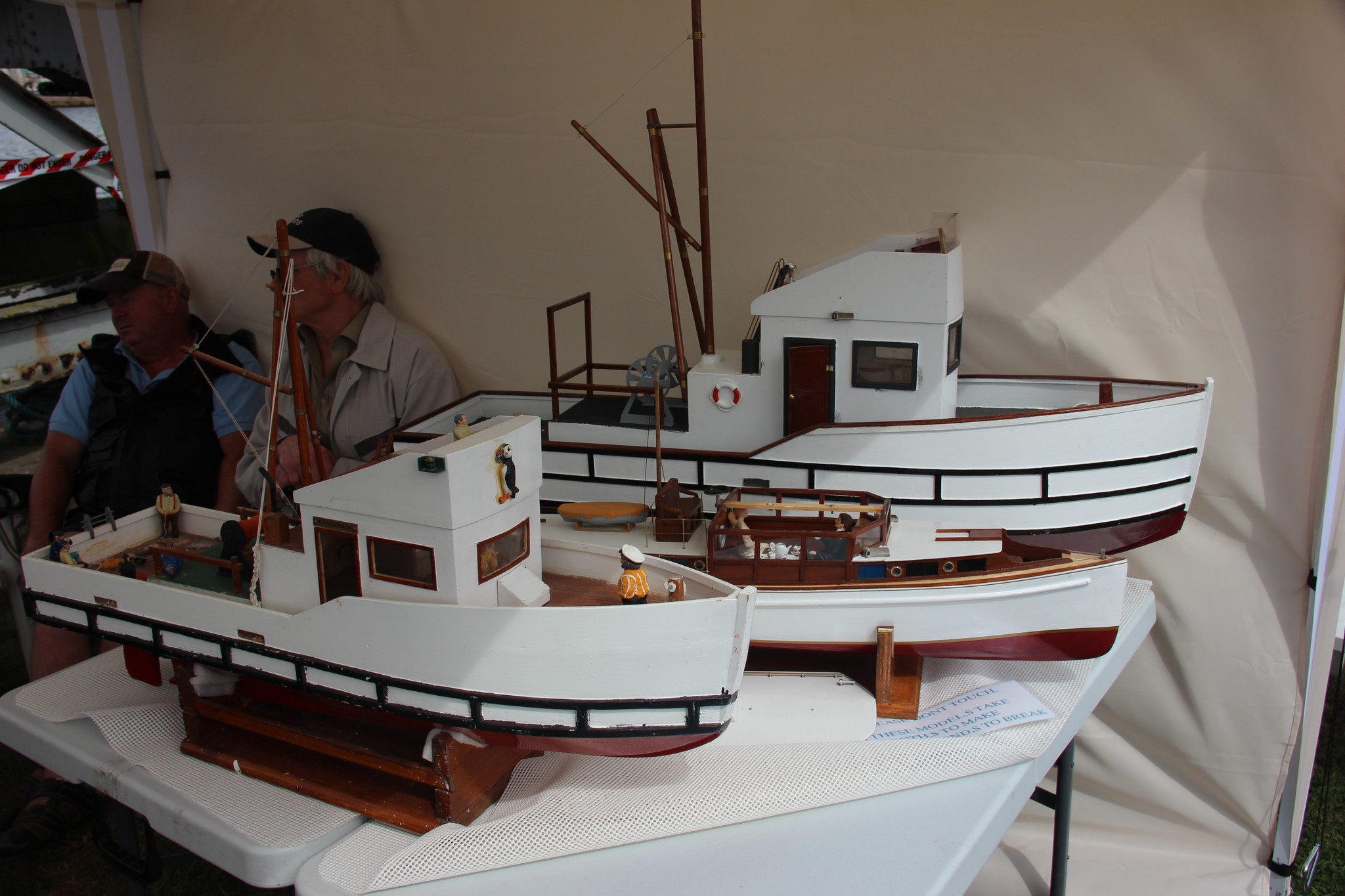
left=2, top=576, right=1159, bottom=896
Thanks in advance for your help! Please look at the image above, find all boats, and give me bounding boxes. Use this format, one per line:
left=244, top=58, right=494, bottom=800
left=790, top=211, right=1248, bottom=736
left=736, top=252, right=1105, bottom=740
left=488, top=370, right=1130, bottom=671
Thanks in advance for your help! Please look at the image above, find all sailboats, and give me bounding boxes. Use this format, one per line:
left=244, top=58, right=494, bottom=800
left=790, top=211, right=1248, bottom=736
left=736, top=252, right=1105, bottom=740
left=395, top=0, right=1215, bottom=557
left=23, top=220, right=758, bottom=736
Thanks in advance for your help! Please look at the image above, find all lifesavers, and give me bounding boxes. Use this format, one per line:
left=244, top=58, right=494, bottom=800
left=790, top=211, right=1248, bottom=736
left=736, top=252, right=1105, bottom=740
left=713, top=382, right=739, bottom=408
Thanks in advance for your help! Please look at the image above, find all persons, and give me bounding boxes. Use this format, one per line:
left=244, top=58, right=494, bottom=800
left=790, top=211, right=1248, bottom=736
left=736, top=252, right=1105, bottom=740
left=20, top=249, right=265, bottom=681
left=234, top=207, right=463, bottom=513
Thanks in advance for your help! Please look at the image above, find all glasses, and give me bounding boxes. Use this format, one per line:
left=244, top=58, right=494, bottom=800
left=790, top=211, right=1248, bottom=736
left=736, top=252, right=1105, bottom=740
left=269, top=261, right=324, bottom=280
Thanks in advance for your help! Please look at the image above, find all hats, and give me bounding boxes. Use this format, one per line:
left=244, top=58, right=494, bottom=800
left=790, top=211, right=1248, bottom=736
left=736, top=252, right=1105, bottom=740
left=75, top=250, right=190, bottom=306
left=246, top=207, right=382, bottom=277
